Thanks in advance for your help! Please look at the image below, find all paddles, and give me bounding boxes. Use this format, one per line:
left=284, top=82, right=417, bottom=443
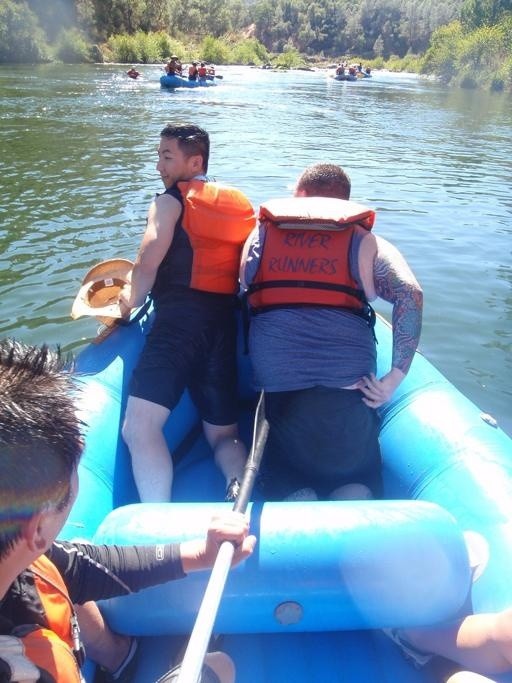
left=206, top=74, right=224, bottom=79
left=173, top=387, right=269, bottom=683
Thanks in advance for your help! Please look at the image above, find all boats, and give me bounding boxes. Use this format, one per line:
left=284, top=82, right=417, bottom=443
left=160, top=74, right=219, bottom=89
left=46, top=270, right=510, bottom=678
left=356, top=72, right=372, bottom=79
left=332, top=73, right=356, bottom=82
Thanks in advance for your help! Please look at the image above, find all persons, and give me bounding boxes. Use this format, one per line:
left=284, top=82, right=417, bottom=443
left=127, top=66, right=139, bottom=78
left=0, top=335, right=259, bottom=683
left=335, top=62, right=372, bottom=76
left=187, top=60, right=198, bottom=81
left=398, top=607, right=512, bottom=683
left=197, top=62, right=207, bottom=80
left=160, top=54, right=187, bottom=77
left=237, top=159, right=426, bottom=502
left=112, top=119, right=257, bottom=507
left=205, top=66, right=215, bottom=81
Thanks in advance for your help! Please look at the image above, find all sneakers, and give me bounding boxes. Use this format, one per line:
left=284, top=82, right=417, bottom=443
left=109, top=634, right=143, bottom=682
left=382, top=626, right=434, bottom=670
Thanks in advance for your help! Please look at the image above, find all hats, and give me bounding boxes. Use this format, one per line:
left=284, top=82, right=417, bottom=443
left=69, top=257, right=141, bottom=330
